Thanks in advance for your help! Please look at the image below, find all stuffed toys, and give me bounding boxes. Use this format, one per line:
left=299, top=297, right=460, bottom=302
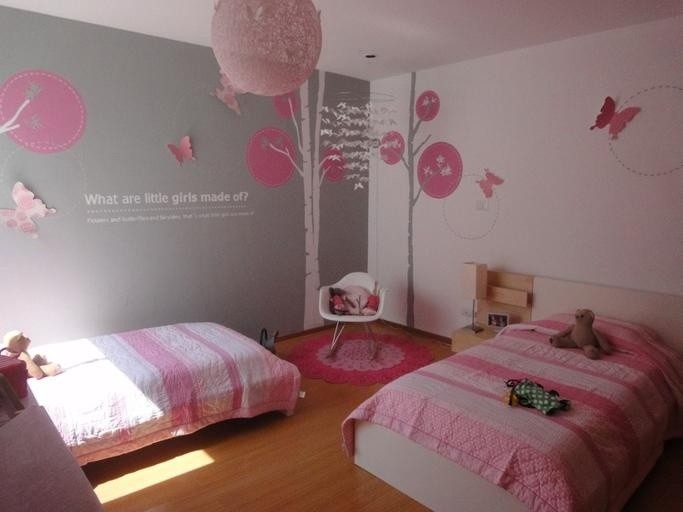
left=549, top=309, right=614, bottom=360
left=6, top=329, right=61, bottom=379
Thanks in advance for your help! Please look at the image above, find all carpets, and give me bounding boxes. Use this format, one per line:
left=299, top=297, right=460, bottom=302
left=286, top=331, right=435, bottom=388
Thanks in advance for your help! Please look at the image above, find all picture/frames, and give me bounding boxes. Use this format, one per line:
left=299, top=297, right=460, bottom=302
left=487, top=311, right=510, bottom=330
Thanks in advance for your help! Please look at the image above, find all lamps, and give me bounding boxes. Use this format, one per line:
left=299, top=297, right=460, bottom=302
left=209, top=2, right=326, bottom=96
left=461, top=259, right=488, bottom=335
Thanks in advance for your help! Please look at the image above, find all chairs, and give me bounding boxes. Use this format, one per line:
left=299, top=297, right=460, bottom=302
left=318, top=270, right=389, bottom=354
left=0, top=321, right=300, bottom=470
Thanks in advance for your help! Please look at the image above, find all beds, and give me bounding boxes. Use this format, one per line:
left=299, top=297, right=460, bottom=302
left=351, top=273, right=680, bottom=512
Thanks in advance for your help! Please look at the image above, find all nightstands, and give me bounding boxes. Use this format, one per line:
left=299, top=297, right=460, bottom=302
left=452, top=270, right=533, bottom=355
